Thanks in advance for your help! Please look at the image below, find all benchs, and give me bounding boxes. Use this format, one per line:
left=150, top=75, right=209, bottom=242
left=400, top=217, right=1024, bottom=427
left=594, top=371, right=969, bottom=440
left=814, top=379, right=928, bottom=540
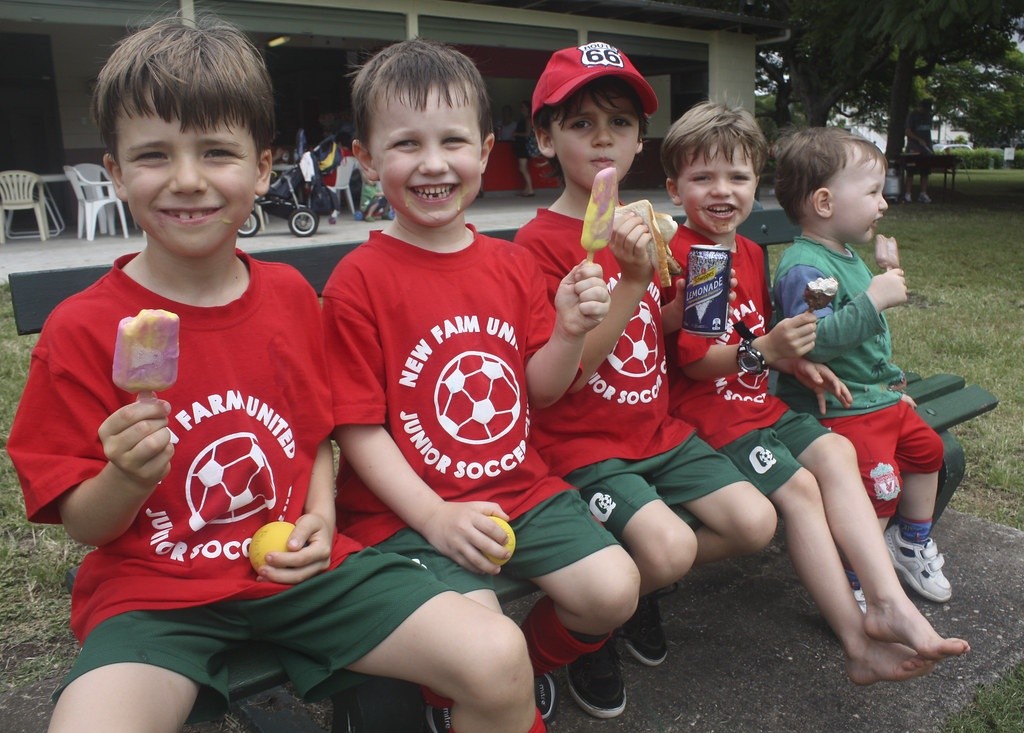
left=5, top=229, right=999, bottom=731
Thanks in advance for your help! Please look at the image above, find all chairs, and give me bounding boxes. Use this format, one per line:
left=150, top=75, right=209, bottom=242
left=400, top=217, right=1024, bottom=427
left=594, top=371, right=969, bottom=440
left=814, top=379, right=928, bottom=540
left=0, top=170, right=51, bottom=244
left=64, top=165, right=130, bottom=244
left=74, top=162, right=140, bottom=236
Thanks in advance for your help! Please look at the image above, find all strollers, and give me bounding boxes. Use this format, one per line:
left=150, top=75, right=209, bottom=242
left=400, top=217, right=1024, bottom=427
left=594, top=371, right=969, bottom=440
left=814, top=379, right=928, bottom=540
left=236, top=134, right=340, bottom=237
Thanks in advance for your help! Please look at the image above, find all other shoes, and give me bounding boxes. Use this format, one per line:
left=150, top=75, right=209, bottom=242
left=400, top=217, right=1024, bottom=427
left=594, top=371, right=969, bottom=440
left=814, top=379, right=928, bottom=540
left=517, top=192, right=535, bottom=197
left=905, top=194, right=912, bottom=203
left=918, top=193, right=931, bottom=203
left=424, top=704, right=450, bottom=733
left=535, top=671, right=556, bottom=721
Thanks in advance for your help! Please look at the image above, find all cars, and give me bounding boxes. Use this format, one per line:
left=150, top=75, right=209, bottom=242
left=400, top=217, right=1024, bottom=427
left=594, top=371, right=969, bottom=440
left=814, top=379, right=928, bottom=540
left=932, top=143, right=976, bottom=152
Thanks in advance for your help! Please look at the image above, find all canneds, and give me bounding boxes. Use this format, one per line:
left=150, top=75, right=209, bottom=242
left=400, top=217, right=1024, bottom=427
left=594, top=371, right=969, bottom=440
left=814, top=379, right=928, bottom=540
left=681, top=241, right=735, bottom=338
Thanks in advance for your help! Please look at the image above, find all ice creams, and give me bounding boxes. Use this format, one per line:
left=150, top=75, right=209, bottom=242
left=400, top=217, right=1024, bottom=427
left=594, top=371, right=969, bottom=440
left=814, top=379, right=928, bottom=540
left=109, top=309, right=183, bottom=399
left=801, top=276, right=837, bottom=316
left=580, top=167, right=619, bottom=269
left=874, top=234, right=899, bottom=271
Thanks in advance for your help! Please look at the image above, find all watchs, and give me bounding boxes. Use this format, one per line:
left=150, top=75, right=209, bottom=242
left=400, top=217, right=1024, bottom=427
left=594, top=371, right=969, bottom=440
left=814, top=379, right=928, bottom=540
left=732, top=319, right=769, bottom=376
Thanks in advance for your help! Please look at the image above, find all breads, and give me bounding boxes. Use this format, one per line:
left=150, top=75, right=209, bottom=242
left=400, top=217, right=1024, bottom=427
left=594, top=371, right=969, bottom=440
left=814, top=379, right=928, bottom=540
left=607, top=198, right=685, bottom=293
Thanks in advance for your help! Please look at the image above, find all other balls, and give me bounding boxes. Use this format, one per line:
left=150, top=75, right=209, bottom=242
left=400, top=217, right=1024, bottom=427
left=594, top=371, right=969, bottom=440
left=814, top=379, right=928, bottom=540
left=480, top=515, right=515, bottom=569
left=249, top=520, right=299, bottom=573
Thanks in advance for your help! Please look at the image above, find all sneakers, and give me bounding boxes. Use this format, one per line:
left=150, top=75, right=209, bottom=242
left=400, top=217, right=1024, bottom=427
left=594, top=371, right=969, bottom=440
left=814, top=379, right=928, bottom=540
left=621, top=595, right=668, bottom=666
left=567, top=638, right=628, bottom=719
left=884, top=524, right=952, bottom=604
left=852, top=590, right=866, bottom=614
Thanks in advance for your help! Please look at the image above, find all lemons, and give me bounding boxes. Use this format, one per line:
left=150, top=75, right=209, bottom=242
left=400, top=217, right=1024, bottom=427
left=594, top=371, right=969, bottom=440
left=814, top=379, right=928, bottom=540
left=248, top=521, right=297, bottom=577
left=478, top=515, right=516, bottom=566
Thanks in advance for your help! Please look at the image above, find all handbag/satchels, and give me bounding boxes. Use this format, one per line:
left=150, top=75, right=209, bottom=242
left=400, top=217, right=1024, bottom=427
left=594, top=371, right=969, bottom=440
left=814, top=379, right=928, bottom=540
left=527, top=133, right=542, bottom=158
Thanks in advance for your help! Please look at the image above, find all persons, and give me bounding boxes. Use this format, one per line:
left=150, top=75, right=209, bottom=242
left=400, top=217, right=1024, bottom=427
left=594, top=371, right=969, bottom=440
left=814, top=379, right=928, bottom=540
left=316, top=43, right=641, bottom=731
left=511, top=102, right=538, bottom=200
left=508, top=41, right=780, bottom=601
left=1, top=13, right=553, bottom=733
left=651, top=99, right=972, bottom=687
left=900, top=96, right=938, bottom=206
left=495, top=104, right=519, bottom=142
left=763, top=122, right=955, bottom=618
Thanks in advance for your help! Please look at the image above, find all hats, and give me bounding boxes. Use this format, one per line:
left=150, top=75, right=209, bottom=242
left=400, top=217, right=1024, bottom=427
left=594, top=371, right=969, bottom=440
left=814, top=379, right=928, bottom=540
left=531, top=42, right=658, bottom=127
left=922, top=100, right=934, bottom=113
